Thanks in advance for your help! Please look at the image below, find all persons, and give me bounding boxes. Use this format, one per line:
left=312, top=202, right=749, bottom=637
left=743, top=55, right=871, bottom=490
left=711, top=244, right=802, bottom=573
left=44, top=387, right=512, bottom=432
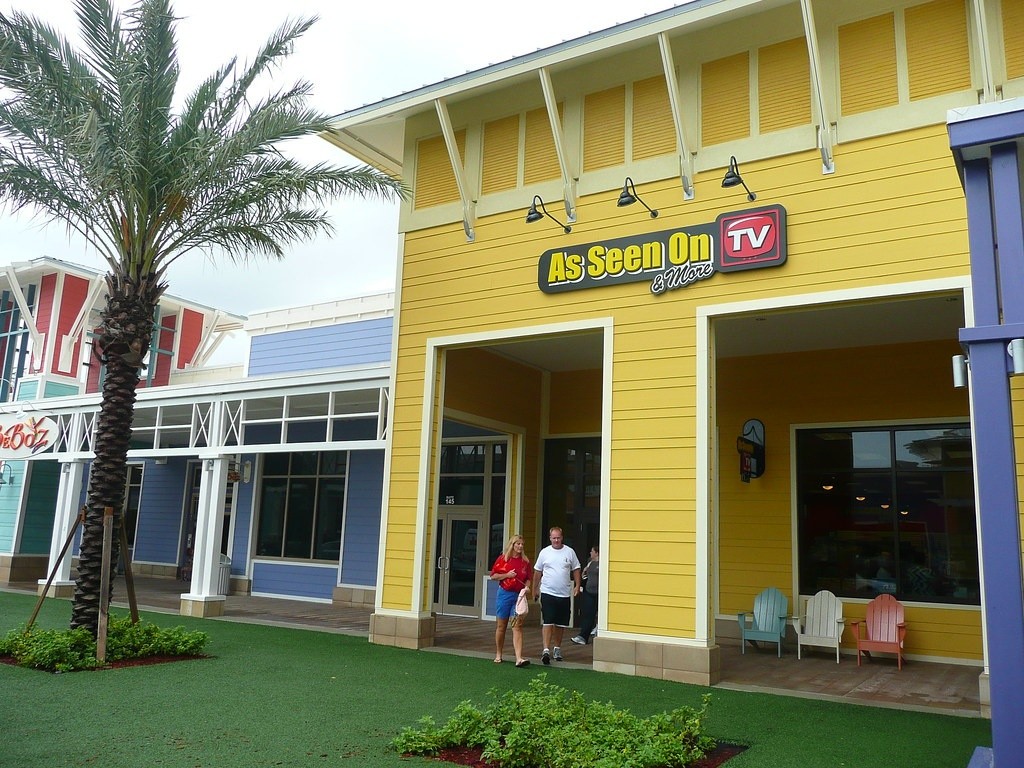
left=490, top=535, right=532, bottom=666
left=571, top=545, right=599, bottom=645
left=531, top=527, right=581, bottom=664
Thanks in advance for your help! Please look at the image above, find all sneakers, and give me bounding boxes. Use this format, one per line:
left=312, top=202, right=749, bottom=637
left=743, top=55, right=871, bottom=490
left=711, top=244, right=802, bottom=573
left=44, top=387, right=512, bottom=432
left=571, top=637, right=585, bottom=645
left=541, top=652, right=551, bottom=665
left=590, top=626, right=598, bottom=635
left=552, top=648, right=563, bottom=661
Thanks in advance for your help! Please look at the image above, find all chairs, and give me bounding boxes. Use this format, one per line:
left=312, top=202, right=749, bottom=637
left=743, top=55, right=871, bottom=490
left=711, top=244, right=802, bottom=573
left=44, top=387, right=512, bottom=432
left=791, top=590, right=847, bottom=664
left=851, top=594, right=909, bottom=670
left=737, top=588, right=789, bottom=658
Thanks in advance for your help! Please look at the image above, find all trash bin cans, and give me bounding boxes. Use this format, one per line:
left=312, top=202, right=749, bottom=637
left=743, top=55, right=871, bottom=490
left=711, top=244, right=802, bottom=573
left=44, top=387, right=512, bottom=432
left=217, top=553, right=232, bottom=595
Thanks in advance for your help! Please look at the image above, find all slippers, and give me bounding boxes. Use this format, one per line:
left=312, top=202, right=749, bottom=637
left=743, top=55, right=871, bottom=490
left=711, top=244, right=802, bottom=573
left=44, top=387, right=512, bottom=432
left=493, top=652, right=502, bottom=663
left=515, top=659, right=530, bottom=667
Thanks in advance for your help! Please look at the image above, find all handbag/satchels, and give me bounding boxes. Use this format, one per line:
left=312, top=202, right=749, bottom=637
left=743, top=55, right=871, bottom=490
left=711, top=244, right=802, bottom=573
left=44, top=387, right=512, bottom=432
left=516, top=586, right=530, bottom=621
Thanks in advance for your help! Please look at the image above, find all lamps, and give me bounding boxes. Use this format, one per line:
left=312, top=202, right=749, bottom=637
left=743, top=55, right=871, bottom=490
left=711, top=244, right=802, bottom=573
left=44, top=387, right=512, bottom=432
left=525, top=195, right=571, bottom=234
left=617, top=177, right=659, bottom=218
left=720, top=156, right=755, bottom=203
left=0, top=464, right=14, bottom=485
